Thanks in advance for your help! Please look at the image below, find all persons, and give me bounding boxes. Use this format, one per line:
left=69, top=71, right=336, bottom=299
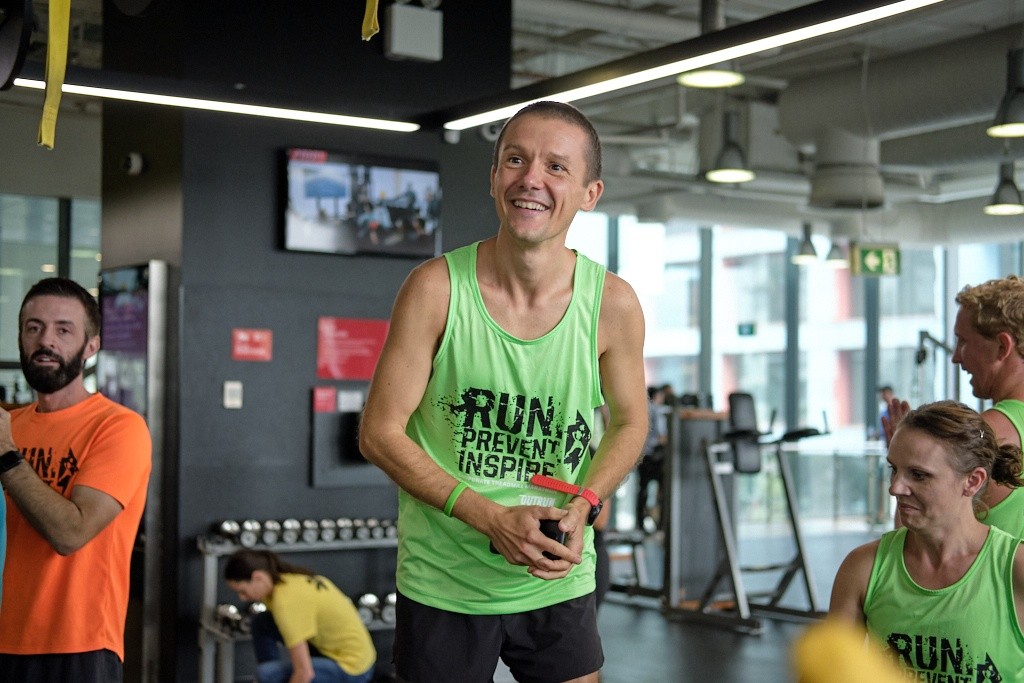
left=224, top=549, right=377, bottom=683
left=0, top=275, right=151, bottom=683
left=359, top=102, right=685, bottom=683
left=826, top=277, right=1024, bottom=683
left=346, top=172, right=442, bottom=250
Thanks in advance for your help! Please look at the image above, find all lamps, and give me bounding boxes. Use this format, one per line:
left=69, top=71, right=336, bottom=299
left=13, top=54, right=419, bottom=136
left=441, top=1, right=944, bottom=132
left=791, top=223, right=817, bottom=265
left=678, top=0, right=746, bottom=90
left=987, top=25, right=1024, bottom=138
left=824, top=230, right=849, bottom=265
left=706, top=96, right=754, bottom=185
left=983, top=139, right=1024, bottom=217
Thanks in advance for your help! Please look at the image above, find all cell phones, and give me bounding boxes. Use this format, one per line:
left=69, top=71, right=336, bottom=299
left=489, top=519, right=567, bottom=561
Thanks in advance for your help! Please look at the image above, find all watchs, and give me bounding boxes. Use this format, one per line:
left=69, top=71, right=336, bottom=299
left=528, top=475, right=604, bottom=527
left=0, top=450, right=25, bottom=474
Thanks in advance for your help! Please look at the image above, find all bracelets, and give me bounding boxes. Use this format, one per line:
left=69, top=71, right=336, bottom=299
left=443, top=482, right=468, bottom=518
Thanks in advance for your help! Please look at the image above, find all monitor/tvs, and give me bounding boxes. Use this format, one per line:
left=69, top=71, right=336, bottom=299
left=280, top=146, right=442, bottom=259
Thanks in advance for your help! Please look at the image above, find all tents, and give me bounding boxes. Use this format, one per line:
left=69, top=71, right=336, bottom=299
left=304, top=177, right=350, bottom=221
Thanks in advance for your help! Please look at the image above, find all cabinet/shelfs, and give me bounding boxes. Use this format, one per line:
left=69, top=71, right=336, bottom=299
left=197, top=535, right=398, bottom=683
